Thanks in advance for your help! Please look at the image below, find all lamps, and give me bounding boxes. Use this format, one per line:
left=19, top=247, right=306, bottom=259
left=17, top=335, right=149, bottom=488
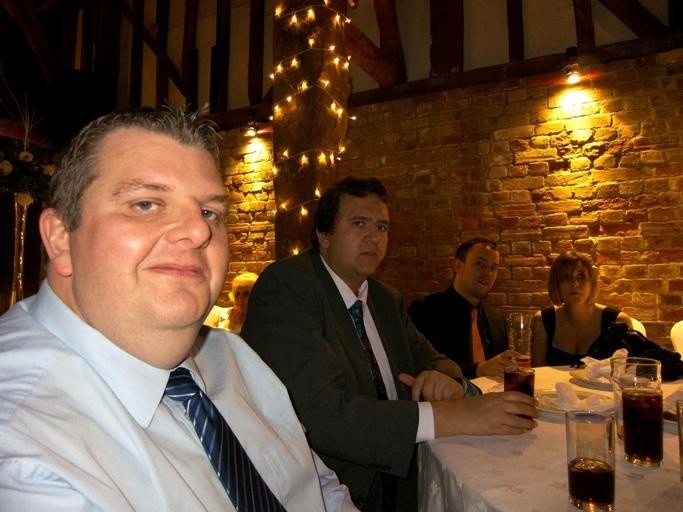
left=245, top=118, right=260, bottom=140
left=565, top=64, right=582, bottom=87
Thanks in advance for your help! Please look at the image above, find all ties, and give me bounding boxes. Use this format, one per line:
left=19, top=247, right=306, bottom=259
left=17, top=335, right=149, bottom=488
left=163, top=365, right=287, bottom=512
left=348, top=298, right=390, bottom=402
left=469, top=307, right=486, bottom=364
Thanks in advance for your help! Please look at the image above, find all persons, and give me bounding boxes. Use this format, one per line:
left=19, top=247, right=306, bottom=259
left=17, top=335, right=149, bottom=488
left=530, top=249, right=633, bottom=368
left=0, top=106, right=358, bottom=512
left=209, top=270, right=259, bottom=337
left=240, top=175, right=541, bottom=512
left=406, top=236, right=523, bottom=381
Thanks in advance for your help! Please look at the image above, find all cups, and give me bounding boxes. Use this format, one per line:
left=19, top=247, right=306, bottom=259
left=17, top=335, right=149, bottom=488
left=611, top=357, right=663, bottom=466
left=509, top=311, right=531, bottom=361
left=565, top=410, right=617, bottom=511
left=503, top=367, right=533, bottom=422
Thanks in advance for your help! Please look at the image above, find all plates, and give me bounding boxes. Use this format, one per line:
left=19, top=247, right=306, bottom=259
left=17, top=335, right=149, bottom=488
left=662, top=387, right=682, bottom=425
left=569, top=369, right=612, bottom=388
left=535, top=388, right=616, bottom=413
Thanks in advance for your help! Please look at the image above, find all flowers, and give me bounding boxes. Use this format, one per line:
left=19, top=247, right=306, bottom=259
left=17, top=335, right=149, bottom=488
left=0, top=71, right=55, bottom=205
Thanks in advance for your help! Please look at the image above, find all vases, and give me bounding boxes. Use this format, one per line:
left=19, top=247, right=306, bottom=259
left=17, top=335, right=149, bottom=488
left=8, top=193, right=30, bottom=306
